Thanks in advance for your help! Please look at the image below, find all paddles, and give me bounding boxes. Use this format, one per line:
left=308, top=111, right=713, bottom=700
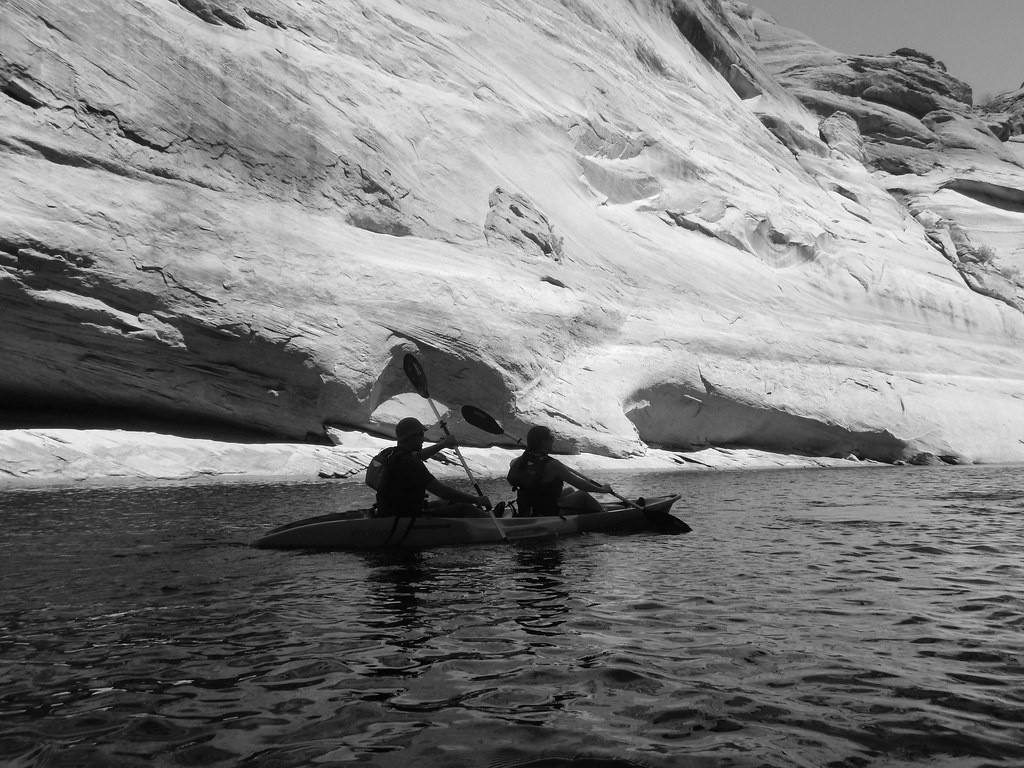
left=403, top=353, right=512, bottom=543
left=461, top=405, right=694, bottom=534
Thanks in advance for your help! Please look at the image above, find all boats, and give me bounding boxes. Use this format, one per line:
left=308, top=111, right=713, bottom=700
left=251, top=492, right=683, bottom=546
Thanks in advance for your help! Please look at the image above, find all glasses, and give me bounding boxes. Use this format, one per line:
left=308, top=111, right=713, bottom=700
left=541, top=435, right=555, bottom=444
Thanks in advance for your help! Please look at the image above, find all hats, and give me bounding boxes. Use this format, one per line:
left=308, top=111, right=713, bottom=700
left=395, top=418, right=429, bottom=444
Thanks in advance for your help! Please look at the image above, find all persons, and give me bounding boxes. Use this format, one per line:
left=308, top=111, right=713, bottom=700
left=508, top=426, right=610, bottom=514
left=376, top=418, right=505, bottom=520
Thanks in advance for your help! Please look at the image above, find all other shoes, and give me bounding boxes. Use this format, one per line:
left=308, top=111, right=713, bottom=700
left=637, top=495, right=645, bottom=506
left=493, top=501, right=505, bottom=518
